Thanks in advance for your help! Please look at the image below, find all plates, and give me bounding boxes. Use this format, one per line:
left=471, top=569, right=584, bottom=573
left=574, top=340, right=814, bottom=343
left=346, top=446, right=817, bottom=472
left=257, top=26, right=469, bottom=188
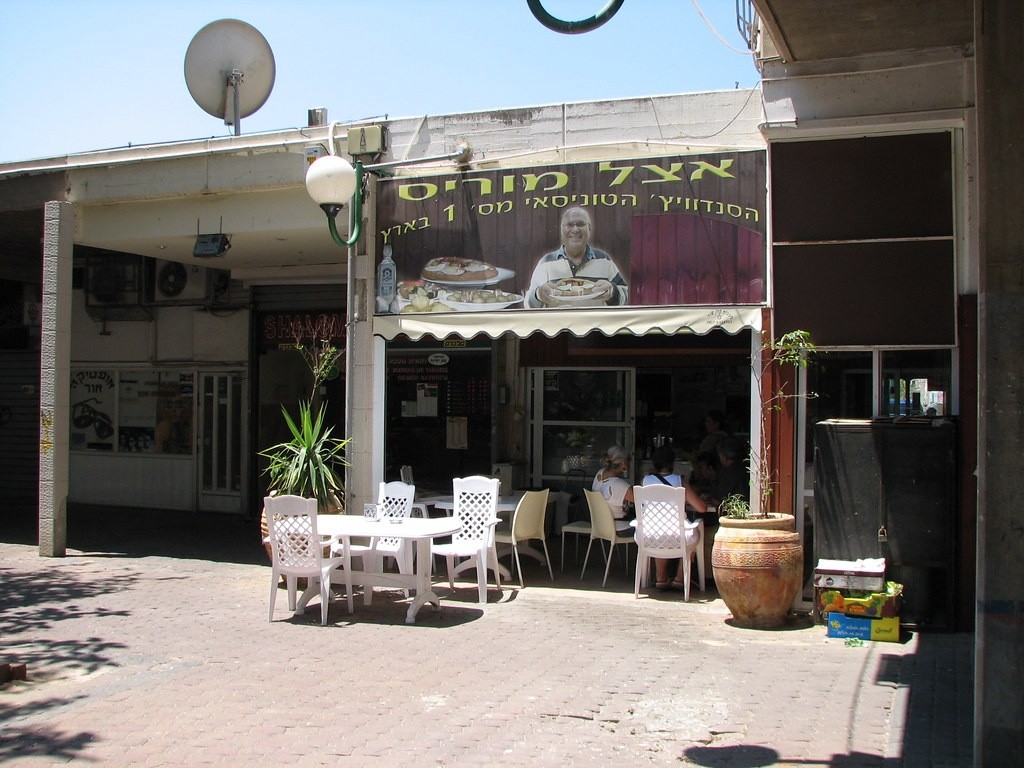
left=544, top=277, right=607, bottom=301
left=397, top=284, right=453, bottom=302
left=419, top=255, right=516, bottom=288
left=441, top=289, right=524, bottom=309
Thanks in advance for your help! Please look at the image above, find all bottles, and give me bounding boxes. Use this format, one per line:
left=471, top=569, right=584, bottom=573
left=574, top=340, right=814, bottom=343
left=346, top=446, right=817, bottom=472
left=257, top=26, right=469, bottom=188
left=378, top=235, right=398, bottom=313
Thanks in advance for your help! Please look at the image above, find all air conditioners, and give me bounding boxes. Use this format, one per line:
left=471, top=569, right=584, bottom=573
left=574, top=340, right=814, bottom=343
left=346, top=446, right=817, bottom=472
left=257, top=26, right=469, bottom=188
left=88, top=264, right=141, bottom=305
left=154, top=259, right=212, bottom=302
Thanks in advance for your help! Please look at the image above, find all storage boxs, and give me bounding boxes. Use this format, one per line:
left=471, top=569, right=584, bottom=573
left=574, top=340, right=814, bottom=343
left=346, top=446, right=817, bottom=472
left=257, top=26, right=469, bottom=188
left=821, top=582, right=903, bottom=617
left=828, top=612, right=899, bottom=643
left=813, top=559, right=886, bottom=591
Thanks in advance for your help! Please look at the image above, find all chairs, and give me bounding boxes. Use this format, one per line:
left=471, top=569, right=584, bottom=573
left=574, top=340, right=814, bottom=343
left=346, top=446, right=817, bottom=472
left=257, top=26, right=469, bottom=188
left=581, top=488, right=635, bottom=588
left=432, top=476, right=503, bottom=603
left=633, top=484, right=705, bottom=602
left=262, top=495, right=353, bottom=626
left=495, top=489, right=554, bottom=589
left=401, top=466, right=460, bottom=571
left=562, top=521, right=623, bottom=574
left=329, top=481, right=415, bottom=606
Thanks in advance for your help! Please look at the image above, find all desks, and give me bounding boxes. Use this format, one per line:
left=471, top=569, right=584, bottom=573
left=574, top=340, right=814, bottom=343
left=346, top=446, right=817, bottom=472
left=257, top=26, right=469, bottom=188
left=630, top=502, right=716, bottom=587
left=435, top=491, right=560, bottom=582
left=273, top=513, right=462, bottom=624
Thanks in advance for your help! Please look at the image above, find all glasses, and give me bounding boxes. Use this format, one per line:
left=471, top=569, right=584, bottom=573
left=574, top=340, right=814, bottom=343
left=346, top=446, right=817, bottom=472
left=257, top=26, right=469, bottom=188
left=563, top=223, right=587, bottom=228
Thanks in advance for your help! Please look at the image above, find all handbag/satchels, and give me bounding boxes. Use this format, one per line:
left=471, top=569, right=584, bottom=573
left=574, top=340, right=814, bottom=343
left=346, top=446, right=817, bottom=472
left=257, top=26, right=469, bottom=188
left=614, top=507, right=636, bottom=521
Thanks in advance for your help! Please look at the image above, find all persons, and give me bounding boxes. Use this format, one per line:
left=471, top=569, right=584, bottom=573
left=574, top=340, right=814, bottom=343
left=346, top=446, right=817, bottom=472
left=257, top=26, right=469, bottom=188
left=591, top=408, right=749, bottom=592
left=523, top=207, right=627, bottom=309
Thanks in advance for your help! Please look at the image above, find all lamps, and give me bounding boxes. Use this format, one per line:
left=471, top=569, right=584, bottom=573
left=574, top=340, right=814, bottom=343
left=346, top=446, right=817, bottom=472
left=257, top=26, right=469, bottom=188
left=306, top=156, right=362, bottom=247
left=193, top=234, right=231, bottom=257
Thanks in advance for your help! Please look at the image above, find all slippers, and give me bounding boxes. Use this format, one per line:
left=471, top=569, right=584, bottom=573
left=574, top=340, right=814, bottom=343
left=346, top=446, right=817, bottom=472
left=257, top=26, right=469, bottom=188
left=655, top=576, right=683, bottom=589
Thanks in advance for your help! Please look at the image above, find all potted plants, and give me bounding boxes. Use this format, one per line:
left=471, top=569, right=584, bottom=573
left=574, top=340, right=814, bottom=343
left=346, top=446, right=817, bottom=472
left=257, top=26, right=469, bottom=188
left=711, top=329, right=820, bottom=627
left=256, top=316, right=356, bottom=589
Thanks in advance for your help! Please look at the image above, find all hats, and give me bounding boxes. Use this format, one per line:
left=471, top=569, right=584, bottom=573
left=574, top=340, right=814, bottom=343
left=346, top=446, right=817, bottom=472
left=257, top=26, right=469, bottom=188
left=651, top=447, right=675, bottom=466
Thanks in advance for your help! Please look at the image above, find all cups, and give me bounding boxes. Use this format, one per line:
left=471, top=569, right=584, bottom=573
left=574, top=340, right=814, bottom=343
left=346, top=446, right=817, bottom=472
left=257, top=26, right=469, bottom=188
left=362, top=504, right=378, bottom=523
left=511, top=408, right=526, bottom=424
